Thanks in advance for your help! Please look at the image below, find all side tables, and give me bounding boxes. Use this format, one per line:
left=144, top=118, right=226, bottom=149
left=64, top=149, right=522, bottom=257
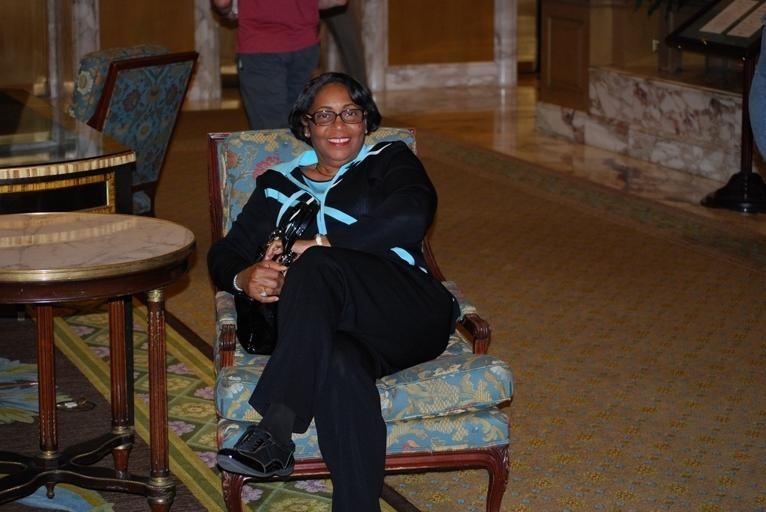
left=0, top=211, right=197, bottom=510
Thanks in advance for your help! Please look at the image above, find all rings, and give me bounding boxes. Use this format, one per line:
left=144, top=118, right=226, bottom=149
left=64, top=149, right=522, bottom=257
left=260, top=287, right=267, bottom=297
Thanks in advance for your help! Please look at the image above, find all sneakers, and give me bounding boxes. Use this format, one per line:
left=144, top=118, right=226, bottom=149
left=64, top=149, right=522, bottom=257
left=216, top=424, right=296, bottom=478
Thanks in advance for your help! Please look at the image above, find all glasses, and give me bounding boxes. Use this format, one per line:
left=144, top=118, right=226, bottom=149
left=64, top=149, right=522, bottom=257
left=305, top=108, right=365, bottom=126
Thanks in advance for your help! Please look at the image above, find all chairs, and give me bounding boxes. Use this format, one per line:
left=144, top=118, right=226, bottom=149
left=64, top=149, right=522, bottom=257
left=204, top=125, right=515, bottom=512
left=67, top=42, right=200, bottom=217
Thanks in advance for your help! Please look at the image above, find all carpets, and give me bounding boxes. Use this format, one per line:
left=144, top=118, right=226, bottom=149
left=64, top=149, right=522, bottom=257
left=0, top=109, right=765, bottom=512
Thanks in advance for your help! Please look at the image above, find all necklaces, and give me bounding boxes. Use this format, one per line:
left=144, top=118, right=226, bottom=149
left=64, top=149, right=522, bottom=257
left=316, top=167, right=334, bottom=177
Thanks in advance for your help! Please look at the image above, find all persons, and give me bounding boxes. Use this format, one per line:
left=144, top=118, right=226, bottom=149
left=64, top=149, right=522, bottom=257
left=205, top=68, right=464, bottom=511
left=210, top=1, right=353, bottom=133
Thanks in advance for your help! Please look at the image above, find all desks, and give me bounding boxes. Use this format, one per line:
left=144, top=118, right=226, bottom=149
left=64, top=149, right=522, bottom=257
left=0, top=88, right=138, bottom=215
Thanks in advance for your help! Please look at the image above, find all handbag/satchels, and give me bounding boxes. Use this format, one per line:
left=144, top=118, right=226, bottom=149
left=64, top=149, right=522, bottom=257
left=233, top=257, right=280, bottom=356
